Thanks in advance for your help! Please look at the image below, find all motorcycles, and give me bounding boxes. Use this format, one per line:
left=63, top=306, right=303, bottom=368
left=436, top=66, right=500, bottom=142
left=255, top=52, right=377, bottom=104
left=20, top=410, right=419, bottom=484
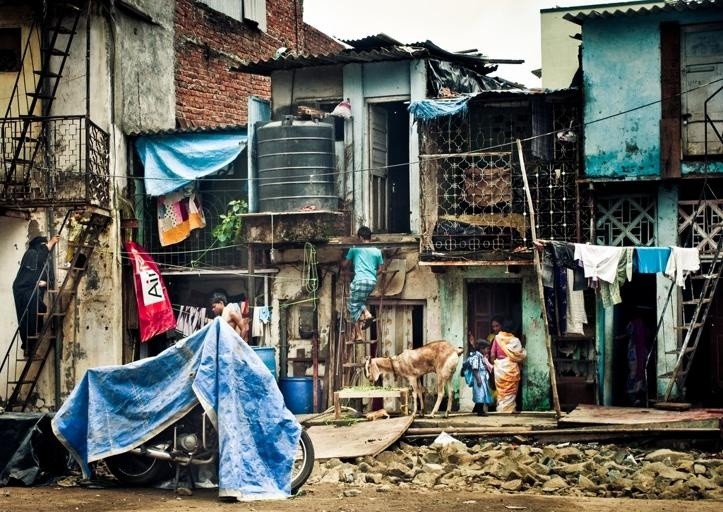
left=105, top=406, right=315, bottom=498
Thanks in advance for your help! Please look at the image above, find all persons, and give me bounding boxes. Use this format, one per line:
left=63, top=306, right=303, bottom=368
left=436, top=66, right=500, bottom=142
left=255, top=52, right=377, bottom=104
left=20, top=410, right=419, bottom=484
left=483, top=315, right=507, bottom=344
left=489, top=319, right=527, bottom=414
left=10, top=233, right=60, bottom=359
left=461, top=339, right=491, bottom=417
left=209, top=287, right=246, bottom=342
left=339, top=226, right=384, bottom=343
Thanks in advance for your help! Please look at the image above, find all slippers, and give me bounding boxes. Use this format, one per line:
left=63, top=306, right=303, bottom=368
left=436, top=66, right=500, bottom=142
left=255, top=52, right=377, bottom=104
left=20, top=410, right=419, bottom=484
left=354, top=339, right=363, bottom=343
left=363, top=317, right=373, bottom=330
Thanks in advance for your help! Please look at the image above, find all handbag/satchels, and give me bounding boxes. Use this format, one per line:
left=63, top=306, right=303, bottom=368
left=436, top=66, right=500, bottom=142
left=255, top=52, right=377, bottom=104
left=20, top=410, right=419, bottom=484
left=462, top=356, right=480, bottom=386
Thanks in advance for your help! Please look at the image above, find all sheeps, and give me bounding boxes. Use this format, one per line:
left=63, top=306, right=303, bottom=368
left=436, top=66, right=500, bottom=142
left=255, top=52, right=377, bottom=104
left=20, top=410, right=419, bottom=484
left=362, top=339, right=465, bottom=418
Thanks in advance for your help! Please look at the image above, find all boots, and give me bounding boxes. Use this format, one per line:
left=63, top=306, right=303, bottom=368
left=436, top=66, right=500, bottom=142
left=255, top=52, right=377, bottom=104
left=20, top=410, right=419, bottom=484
left=472, top=404, right=487, bottom=416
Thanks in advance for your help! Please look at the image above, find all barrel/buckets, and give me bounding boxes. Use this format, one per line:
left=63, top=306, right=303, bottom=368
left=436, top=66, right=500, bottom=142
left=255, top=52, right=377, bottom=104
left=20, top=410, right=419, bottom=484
left=254, top=114, right=340, bottom=214
left=251, top=346, right=277, bottom=380
left=279, top=378, right=322, bottom=416
